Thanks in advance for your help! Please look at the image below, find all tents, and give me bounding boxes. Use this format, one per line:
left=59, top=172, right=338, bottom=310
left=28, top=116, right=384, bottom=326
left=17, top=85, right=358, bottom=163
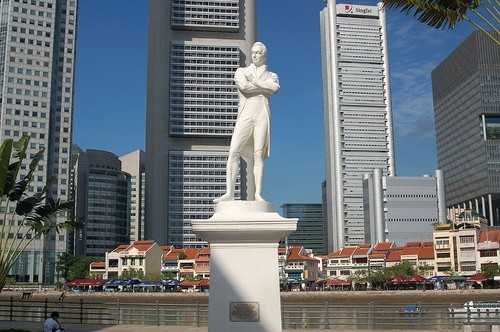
left=64, top=273, right=497, bottom=295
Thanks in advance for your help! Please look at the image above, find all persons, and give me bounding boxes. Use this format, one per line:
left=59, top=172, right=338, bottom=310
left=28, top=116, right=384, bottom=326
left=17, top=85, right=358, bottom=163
left=212, top=42, right=280, bottom=203
left=43, top=312, right=64, bottom=332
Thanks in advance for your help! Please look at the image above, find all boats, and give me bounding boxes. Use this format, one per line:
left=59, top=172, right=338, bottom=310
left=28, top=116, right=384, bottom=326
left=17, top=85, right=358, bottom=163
left=395, top=305, right=431, bottom=319
left=447, top=299, right=500, bottom=318
